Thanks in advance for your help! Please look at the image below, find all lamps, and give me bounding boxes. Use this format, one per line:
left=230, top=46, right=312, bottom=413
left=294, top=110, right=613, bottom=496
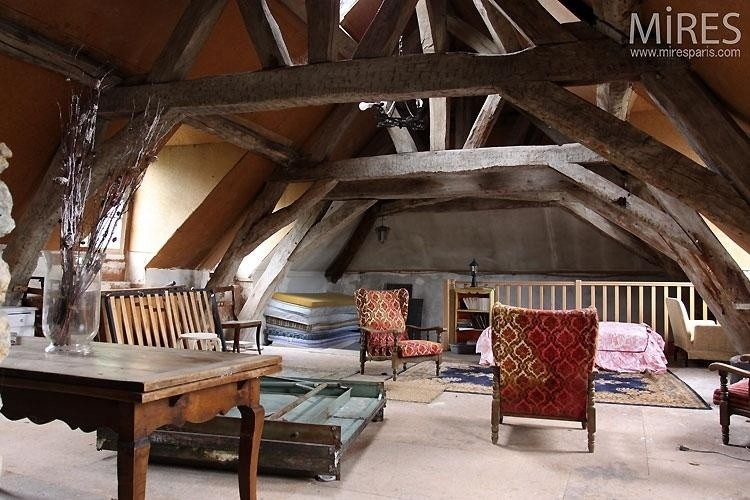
left=469, top=258, right=479, bottom=287
left=374, top=214, right=392, bottom=244
left=358, top=32, right=427, bottom=132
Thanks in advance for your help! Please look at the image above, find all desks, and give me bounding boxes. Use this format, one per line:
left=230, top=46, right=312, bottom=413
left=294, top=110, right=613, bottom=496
left=0, top=335, right=282, bottom=500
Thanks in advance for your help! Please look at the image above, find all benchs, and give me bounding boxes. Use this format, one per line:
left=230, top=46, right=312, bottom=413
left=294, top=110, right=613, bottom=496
left=473, top=318, right=671, bottom=375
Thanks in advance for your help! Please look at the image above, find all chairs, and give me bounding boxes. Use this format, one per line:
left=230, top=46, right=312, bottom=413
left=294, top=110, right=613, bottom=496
left=356, top=286, right=444, bottom=381
left=209, top=284, right=262, bottom=356
left=666, top=297, right=739, bottom=363
left=489, top=300, right=597, bottom=455
left=710, top=353, right=750, bottom=447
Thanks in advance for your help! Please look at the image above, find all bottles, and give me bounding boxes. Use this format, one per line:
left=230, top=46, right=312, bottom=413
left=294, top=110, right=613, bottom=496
left=464, top=284, right=467, bottom=289
left=439, top=327, right=448, bottom=352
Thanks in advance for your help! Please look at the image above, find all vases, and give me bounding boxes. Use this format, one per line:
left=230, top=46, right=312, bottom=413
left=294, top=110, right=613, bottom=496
left=38, top=247, right=106, bottom=359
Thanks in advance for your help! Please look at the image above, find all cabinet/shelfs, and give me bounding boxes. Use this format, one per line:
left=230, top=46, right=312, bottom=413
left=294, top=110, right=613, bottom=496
left=382, top=281, right=425, bottom=341
left=1, top=306, right=38, bottom=335
left=449, top=285, right=496, bottom=354
left=23, top=275, right=47, bottom=338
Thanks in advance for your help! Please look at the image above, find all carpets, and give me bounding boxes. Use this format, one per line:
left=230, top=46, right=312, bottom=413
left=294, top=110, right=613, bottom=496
left=383, top=349, right=712, bottom=412
left=266, top=362, right=363, bottom=383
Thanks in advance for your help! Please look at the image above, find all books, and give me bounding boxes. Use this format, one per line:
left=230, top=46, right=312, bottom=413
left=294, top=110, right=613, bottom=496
left=456, top=296, right=489, bottom=329
